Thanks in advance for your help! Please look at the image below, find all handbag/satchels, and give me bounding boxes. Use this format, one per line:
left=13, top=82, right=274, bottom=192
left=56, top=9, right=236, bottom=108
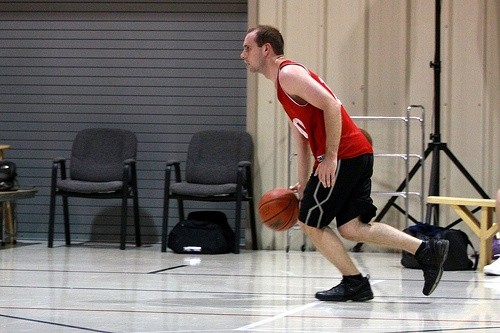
left=168, top=211, right=233, bottom=254
left=399, top=223, right=478, bottom=271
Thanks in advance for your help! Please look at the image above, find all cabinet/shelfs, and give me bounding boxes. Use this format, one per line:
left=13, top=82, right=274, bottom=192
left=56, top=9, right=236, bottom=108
left=351, top=106, right=426, bottom=238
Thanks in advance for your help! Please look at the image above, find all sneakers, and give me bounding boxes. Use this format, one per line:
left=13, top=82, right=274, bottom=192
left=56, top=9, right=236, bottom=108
left=416, top=239, right=450, bottom=296
left=315, top=275, right=374, bottom=301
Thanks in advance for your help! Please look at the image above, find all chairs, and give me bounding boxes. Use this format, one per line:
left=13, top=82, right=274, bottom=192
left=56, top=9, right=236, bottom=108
left=48, top=128, right=141, bottom=250
left=161, top=129, right=258, bottom=254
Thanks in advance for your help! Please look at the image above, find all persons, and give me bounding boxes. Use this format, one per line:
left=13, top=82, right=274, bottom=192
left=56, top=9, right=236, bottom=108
left=239, top=25, right=450, bottom=301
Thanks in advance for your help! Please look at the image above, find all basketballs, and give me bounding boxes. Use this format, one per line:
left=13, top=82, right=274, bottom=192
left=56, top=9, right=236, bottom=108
left=259, top=186, right=300, bottom=232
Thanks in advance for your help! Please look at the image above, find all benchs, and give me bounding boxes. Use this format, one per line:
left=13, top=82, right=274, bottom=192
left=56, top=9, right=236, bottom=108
left=426, top=196, right=498, bottom=273
left=0, top=189, right=38, bottom=247
